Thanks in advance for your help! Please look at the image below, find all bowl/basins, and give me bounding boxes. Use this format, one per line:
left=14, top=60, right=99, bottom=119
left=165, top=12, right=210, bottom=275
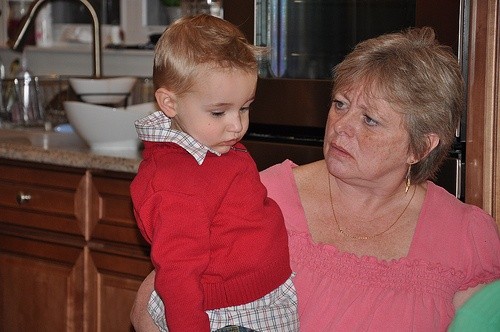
left=62, top=100, right=164, bottom=151
left=66, top=76, right=138, bottom=105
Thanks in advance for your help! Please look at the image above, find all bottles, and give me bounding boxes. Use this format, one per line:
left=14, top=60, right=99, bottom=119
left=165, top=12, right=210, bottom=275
left=8, top=0, right=36, bottom=51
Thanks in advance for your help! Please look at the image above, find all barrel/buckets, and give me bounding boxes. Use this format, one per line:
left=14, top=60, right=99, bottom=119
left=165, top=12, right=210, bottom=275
left=0, top=76, right=60, bottom=123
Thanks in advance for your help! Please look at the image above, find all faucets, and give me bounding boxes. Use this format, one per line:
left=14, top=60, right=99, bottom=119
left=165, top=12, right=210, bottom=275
left=8, top=0, right=103, bottom=77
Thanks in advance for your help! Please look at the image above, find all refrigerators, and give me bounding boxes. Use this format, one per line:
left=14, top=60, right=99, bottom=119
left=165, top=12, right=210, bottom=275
left=219, top=0, right=469, bottom=200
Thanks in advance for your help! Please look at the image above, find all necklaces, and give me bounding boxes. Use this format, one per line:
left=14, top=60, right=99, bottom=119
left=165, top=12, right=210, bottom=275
left=327, top=162, right=417, bottom=239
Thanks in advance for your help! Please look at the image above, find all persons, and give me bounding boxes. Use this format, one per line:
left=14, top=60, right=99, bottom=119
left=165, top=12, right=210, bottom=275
left=130, top=27, right=500, bottom=332
left=128, top=15, right=300, bottom=332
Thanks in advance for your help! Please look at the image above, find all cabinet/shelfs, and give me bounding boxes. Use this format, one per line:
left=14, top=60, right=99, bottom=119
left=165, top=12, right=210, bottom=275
left=220, top=0, right=472, bottom=202
left=1, top=157, right=151, bottom=332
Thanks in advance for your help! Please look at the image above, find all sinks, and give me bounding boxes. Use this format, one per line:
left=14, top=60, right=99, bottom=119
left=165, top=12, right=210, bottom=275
left=8, top=108, right=160, bottom=135
